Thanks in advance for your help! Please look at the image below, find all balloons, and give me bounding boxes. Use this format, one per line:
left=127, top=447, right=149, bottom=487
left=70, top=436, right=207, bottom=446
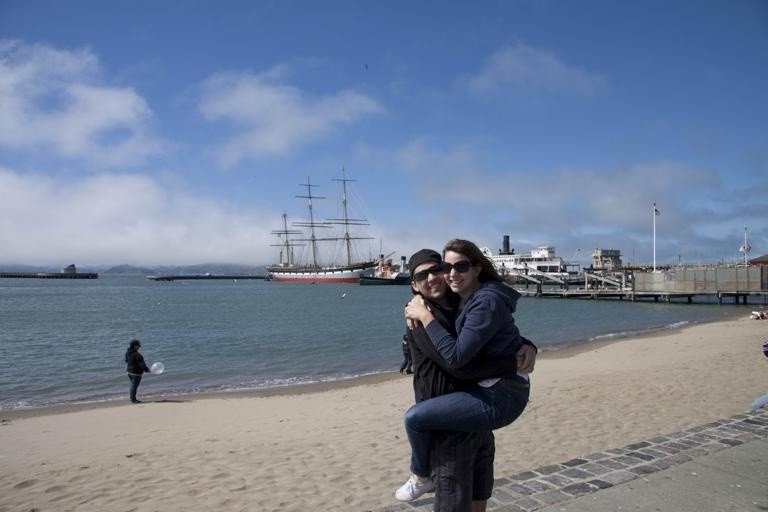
left=150, top=361, right=165, bottom=374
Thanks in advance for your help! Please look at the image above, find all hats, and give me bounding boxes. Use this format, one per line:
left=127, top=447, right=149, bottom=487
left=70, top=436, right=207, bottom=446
left=408, top=249, right=443, bottom=278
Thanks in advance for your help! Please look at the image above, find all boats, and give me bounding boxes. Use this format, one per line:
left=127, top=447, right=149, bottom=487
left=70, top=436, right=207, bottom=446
left=480, top=235, right=586, bottom=285
left=358, top=256, right=412, bottom=287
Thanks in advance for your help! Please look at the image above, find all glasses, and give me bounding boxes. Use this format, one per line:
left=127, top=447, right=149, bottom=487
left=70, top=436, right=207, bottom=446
left=413, top=265, right=442, bottom=281
left=441, top=259, right=472, bottom=273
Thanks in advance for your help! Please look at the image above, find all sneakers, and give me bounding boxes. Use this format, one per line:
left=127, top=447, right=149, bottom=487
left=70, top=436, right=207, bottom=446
left=395, top=476, right=435, bottom=502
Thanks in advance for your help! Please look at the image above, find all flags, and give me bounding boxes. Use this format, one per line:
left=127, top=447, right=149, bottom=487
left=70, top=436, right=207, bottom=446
left=655, top=208, right=661, bottom=217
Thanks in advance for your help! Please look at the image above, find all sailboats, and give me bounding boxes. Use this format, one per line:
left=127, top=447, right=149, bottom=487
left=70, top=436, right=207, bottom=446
left=265, top=166, right=396, bottom=284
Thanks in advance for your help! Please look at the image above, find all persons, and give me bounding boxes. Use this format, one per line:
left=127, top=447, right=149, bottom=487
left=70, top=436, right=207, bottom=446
left=393, top=237, right=531, bottom=502
left=124, top=339, right=151, bottom=404
left=750, top=310, right=768, bottom=321
left=398, top=333, right=414, bottom=374
left=402, top=248, right=539, bottom=512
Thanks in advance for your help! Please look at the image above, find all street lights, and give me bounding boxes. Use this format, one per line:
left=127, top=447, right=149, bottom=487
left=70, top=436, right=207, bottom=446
left=652, top=201, right=661, bottom=274
left=743, top=226, right=748, bottom=266
